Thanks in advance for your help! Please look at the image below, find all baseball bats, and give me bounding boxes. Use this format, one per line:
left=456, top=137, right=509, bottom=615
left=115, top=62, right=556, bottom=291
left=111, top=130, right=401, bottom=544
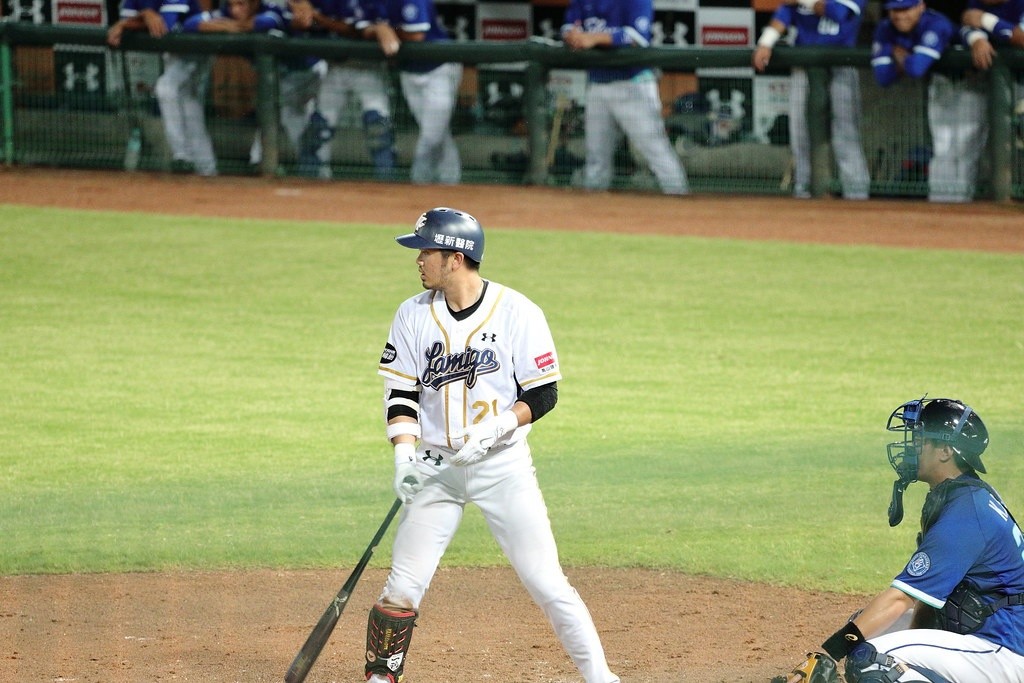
left=284, top=475, right=419, bottom=683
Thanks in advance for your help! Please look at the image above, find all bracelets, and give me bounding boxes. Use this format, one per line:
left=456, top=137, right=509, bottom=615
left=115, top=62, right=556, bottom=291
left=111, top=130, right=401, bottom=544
left=799, top=0, right=818, bottom=12
left=982, top=12, right=999, bottom=31
left=757, top=26, right=780, bottom=48
left=967, top=30, right=988, bottom=45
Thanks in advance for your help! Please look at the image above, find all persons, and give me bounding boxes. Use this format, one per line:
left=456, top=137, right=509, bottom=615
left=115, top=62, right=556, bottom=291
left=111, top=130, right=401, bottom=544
left=561, top=0, right=689, bottom=194
left=958, top=0, right=1024, bottom=197
left=106, top=0, right=465, bottom=183
left=363, top=206, right=621, bottom=683
left=772, top=392, right=1024, bottom=683
left=870, top=0, right=990, bottom=202
left=751, top=0, right=870, bottom=199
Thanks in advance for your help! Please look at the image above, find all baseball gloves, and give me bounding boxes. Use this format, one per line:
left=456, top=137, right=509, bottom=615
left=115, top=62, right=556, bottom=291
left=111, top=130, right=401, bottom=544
left=771, top=652, right=844, bottom=683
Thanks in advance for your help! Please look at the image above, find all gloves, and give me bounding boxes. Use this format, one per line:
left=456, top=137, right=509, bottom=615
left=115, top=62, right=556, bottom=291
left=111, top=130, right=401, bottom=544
left=394, top=462, right=424, bottom=505
left=448, top=415, right=507, bottom=467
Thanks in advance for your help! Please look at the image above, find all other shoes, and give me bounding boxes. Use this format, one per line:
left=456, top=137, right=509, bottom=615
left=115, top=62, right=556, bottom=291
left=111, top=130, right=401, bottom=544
left=170, top=158, right=195, bottom=175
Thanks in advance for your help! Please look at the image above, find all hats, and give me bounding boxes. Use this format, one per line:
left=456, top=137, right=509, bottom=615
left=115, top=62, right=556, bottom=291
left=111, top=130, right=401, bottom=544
left=885, top=0, right=922, bottom=11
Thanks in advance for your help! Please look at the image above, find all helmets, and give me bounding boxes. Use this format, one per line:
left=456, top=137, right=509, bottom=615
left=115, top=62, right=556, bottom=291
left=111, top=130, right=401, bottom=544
left=394, top=207, right=485, bottom=263
left=922, top=399, right=990, bottom=473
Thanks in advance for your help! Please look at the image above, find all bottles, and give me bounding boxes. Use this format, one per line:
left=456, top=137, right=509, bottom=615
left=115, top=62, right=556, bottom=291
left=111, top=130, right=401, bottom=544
left=124, top=130, right=141, bottom=172
left=573, top=20, right=583, bottom=52
